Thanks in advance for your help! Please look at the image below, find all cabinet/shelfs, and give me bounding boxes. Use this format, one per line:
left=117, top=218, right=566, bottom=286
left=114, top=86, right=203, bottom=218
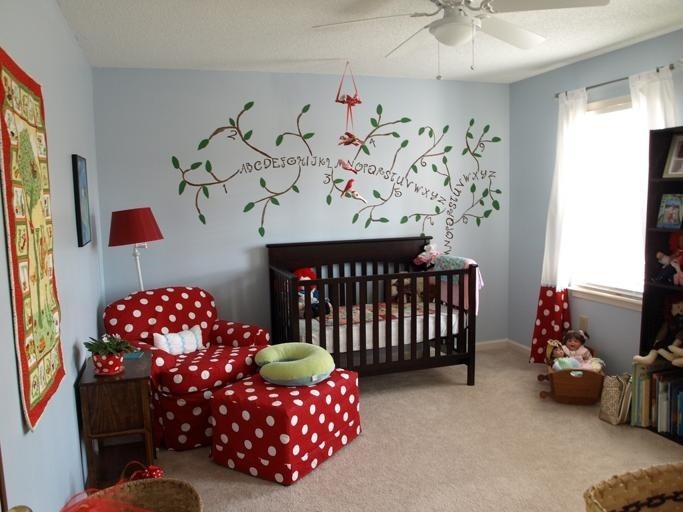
left=639, top=125, right=683, bottom=445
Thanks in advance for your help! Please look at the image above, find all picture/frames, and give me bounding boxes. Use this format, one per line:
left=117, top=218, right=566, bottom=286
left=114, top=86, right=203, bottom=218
left=656, top=194, right=683, bottom=230
left=662, top=134, right=683, bottom=179
left=72, top=154, right=91, bottom=247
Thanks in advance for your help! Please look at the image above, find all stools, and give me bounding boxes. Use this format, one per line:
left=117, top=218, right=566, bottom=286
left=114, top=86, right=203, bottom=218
left=210, top=366, right=362, bottom=487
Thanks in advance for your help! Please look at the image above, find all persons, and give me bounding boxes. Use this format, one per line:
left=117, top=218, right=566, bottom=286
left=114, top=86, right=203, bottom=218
left=546, top=328, right=607, bottom=376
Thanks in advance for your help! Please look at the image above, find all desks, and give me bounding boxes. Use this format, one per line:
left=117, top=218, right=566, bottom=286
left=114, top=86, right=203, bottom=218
left=77, top=351, right=157, bottom=490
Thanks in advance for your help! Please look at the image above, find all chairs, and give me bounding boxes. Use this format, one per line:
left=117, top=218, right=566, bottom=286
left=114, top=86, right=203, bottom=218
left=104, top=286, right=270, bottom=451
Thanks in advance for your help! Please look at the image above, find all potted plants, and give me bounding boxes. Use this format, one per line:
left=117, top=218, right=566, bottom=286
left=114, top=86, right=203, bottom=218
left=83, top=334, right=131, bottom=376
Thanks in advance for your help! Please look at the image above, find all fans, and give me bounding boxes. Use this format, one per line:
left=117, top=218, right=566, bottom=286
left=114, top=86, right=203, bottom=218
left=312, top=0, right=610, bottom=81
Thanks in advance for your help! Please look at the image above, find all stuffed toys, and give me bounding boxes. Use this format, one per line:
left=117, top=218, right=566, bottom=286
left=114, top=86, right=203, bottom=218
left=293, top=266, right=331, bottom=320
left=632, top=249, right=683, bottom=368
left=390, top=270, right=424, bottom=303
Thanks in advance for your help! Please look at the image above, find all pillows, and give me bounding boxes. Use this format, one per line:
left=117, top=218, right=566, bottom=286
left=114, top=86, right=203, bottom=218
left=152, top=324, right=204, bottom=356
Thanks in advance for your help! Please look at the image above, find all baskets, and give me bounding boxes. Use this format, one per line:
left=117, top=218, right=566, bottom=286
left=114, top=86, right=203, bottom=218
left=59, top=476, right=203, bottom=511
left=582, top=461, right=683, bottom=512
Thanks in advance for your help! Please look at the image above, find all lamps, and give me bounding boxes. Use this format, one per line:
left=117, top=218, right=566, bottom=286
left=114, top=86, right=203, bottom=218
left=429, top=7, right=482, bottom=81
left=107, top=208, right=164, bottom=292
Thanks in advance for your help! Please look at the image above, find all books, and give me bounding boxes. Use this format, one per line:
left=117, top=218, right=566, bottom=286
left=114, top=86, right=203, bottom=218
left=630, top=359, right=683, bottom=436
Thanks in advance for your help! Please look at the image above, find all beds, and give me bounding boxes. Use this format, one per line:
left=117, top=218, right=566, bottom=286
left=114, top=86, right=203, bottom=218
left=265, top=236, right=478, bottom=385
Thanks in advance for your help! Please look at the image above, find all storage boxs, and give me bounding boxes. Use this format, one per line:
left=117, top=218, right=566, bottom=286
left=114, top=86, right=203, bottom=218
left=537, top=347, right=604, bottom=405
